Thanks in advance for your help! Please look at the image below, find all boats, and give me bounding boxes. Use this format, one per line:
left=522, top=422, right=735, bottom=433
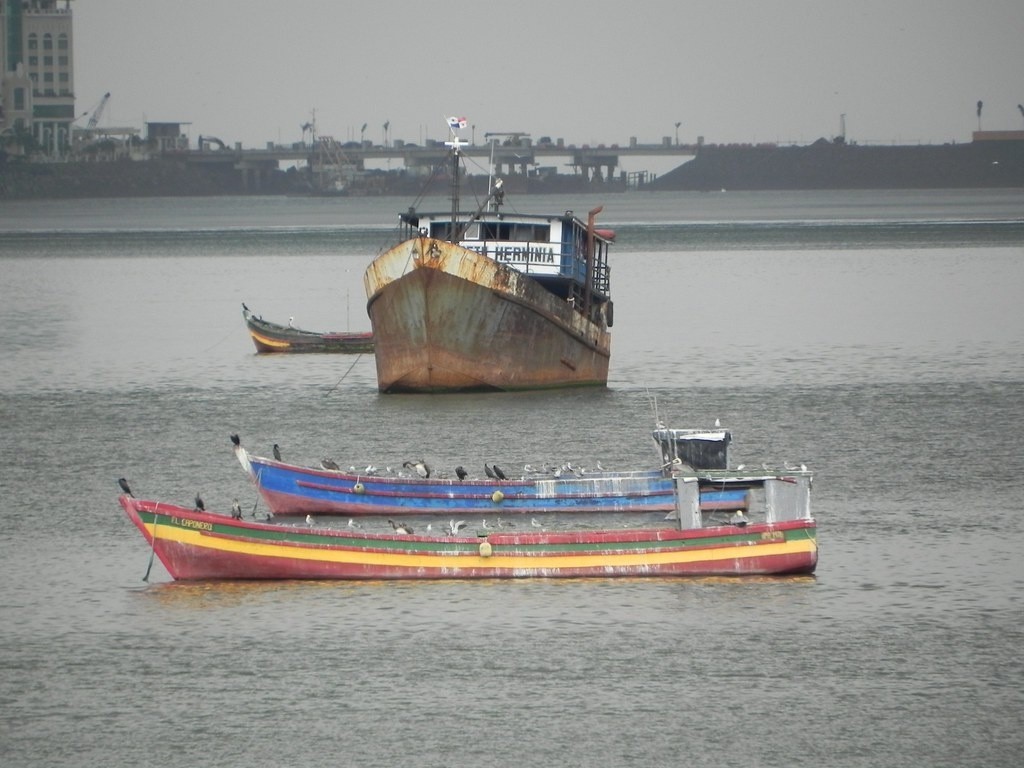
left=243, top=302, right=378, bottom=357
left=233, top=431, right=803, bottom=516
left=360, top=114, right=617, bottom=392
left=118, top=477, right=820, bottom=583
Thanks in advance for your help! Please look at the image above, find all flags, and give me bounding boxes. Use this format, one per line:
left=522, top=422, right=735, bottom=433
left=447, top=117, right=466, bottom=129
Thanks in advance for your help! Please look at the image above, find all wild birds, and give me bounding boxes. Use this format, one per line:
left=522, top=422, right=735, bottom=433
left=273, top=443, right=282, bottom=462
left=304, top=515, right=317, bottom=529
left=242, top=302, right=271, bottom=331
left=229, top=433, right=240, bottom=447
left=524, top=459, right=611, bottom=480
left=230, top=498, right=243, bottom=521
left=455, top=466, right=469, bottom=480
left=192, top=490, right=207, bottom=514
left=402, top=459, right=430, bottom=479
left=318, top=456, right=396, bottom=477
left=714, top=418, right=721, bottom=429
left=484, top=462, right=509, bottom=481
left=388, top=517, right=516, bottom=536
left=655, top=421, right=667, bottom=430
left=116, top=477, right=134, bottom=497
left=530, top=516, right=545, bottom=532
left=288, top=316, right=299, bottom=330
left=736, top=459, right=794, bottom=471
left=347, top=517, right=364, bottom=534
